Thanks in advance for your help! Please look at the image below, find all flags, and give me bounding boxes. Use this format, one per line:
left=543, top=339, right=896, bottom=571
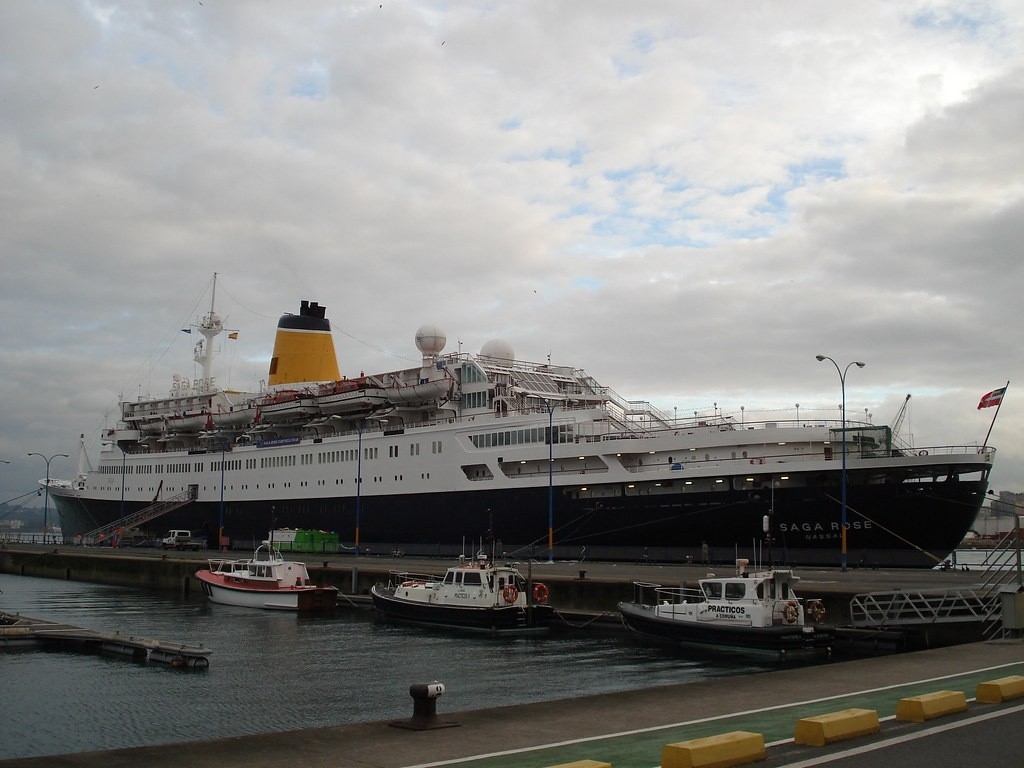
left=978, top=387, right=1006, bottom=410
left=205, top=413, right=213, bottom=429
left=183, top=329, right=191, bottom=334
left=255, top=407, right=262, bottom=422
left=228, top=333, right=237, bottom=339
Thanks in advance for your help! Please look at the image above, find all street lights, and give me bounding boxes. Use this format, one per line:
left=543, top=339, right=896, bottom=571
left=816, top=355, right=865, bottom=572
left=101, top=441, right=149, bottom=549
left=199, top=430, right=249, bottom=552
left=27, top=453, right=71, bottom=546
left=331, top=412, right=389, bottom=559
left=521, top=389, right=580, bottom=564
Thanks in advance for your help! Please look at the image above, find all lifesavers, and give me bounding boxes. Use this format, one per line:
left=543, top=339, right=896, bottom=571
left=501, top=583, right=520, bottom=604
left=806, top=599, right=827, bottom=624
left=531, top=582, right=551, bottom=603
left=781, top=600, right=802, bottom=625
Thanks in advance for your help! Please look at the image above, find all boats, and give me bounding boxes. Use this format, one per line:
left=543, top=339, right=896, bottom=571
left=369, top=535, right=557, bottom=639
left=615, top=537, right=859, bottom=668
left=194, top=539, right=339, bottom=613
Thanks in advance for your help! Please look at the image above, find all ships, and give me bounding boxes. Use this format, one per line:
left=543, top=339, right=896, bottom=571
left=35, top=270, right=1011, bottom=571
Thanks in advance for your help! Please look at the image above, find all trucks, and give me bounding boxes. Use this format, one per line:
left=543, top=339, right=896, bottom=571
left=162, top=529, right=202, bottom=552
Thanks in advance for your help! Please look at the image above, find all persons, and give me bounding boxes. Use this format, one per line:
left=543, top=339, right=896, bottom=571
left=74, top=528, right=120, bottom=548
left=295, top=576, right=310, bottom=586
